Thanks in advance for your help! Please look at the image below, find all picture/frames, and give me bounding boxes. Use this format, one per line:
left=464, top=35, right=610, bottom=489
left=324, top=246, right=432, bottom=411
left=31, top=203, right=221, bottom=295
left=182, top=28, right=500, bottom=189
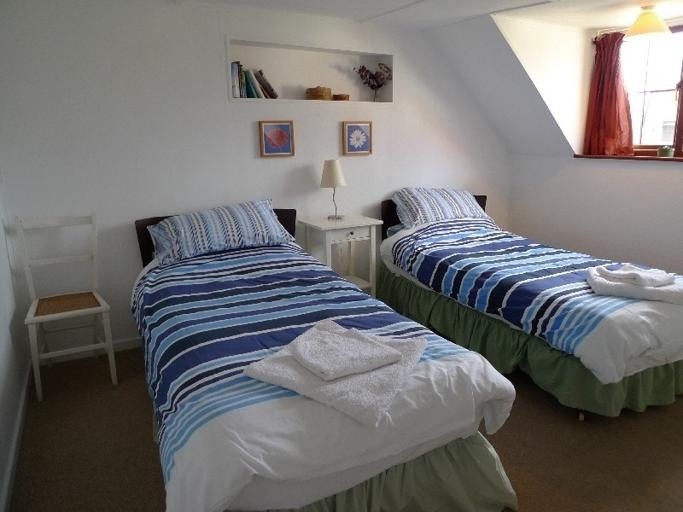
left=259, top=120, right=295, bottom=158
left=343, top=121, right=373, bottom=156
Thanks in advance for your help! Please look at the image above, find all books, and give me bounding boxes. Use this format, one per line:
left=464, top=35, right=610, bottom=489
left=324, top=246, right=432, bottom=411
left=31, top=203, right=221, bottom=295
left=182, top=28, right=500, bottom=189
left=231, top=59, right=278, bottom=99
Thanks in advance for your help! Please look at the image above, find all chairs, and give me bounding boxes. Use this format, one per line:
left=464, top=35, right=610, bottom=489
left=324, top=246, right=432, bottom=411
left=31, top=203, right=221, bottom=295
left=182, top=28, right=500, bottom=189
left=15, top=213, right=119, bottom=402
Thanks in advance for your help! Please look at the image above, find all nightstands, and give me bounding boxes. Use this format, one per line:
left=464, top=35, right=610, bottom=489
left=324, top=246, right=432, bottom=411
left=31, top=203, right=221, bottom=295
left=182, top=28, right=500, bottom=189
left=298, top=213, right=384, bottom=298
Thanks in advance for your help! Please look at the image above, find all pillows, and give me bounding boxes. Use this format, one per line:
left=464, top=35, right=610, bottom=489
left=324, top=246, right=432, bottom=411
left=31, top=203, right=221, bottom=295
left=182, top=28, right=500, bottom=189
left=146, top=199, right=296, bottom=268
left=392, top=187, right=487, bottom=228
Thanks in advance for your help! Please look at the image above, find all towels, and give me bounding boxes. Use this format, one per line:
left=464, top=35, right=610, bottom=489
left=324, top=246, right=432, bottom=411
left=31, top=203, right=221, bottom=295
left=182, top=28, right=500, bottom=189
left=586, top=262, right=683, bottom=304
left=244, top=318, right=428, bottom=428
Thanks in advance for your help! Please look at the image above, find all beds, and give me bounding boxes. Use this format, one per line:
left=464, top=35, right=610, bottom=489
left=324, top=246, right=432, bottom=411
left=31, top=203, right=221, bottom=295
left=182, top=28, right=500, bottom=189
left=376, top=195, right=683, bottom=421
left=130, top=209, right=519, bottom=512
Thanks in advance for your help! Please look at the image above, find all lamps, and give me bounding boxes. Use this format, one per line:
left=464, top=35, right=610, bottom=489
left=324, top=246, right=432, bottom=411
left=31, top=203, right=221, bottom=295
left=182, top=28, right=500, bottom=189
left=321, top=159, right=347, bottom=219
left=627, top=4, right=673, bottom=37
